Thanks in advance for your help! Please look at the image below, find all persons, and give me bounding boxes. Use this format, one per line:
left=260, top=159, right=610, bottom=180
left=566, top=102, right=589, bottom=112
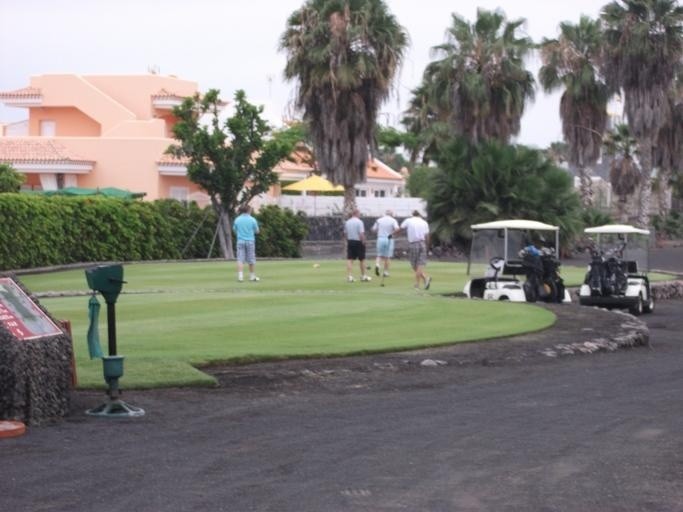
left=341, top=208, right=371, bottom=283
left=368, top=209, right=398, bottom=277
left=395, top=208, right=433, bottom=291
left=231, top=204, right=260, bottom=283
left=516, top=236, right=551, bottom=257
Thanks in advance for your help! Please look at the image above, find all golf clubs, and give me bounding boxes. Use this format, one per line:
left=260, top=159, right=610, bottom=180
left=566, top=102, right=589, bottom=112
left=380, top=238, right=390, bottom=287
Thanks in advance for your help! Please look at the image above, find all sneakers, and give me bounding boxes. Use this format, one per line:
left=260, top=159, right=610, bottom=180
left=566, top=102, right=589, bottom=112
left=347, top=264, right=389, bottom=282
left=425, top=276, right=432, bottom=290
left=237, top=275, right=260, bottom=282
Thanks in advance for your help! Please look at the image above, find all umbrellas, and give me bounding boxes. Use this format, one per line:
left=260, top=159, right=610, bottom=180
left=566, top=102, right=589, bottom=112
left=282, top=173, right=344, bottom=216
